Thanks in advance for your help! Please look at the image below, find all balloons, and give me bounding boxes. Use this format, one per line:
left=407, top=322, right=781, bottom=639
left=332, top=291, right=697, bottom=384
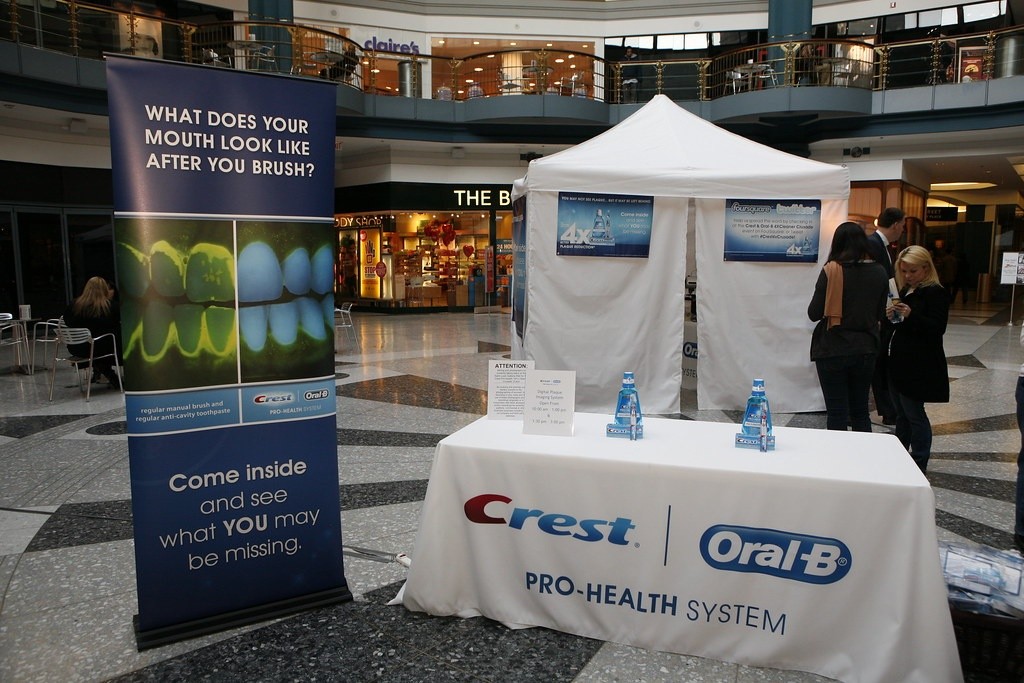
left=464, top=246, right=474, bottom=258
left=424, top=219, right=456, bottom=247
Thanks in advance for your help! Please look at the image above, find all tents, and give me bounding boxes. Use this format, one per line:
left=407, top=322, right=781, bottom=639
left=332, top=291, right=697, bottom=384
left=514, top=92, right=851, bottom=413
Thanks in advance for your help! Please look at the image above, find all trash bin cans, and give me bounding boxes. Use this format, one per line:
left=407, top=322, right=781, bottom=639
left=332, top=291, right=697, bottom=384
left=975, top=272, right=993, bottom=304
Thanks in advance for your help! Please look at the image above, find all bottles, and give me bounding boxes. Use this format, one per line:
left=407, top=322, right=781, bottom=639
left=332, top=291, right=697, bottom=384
left=742, top=379, right=772, bottom=437
left=888, top=295, right=901, bottom=323
left=616, top=372, right=642, bottom=424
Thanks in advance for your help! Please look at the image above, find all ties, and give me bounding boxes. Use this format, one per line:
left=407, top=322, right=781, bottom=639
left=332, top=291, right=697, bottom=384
left=888, top=245, right=894, bottom=261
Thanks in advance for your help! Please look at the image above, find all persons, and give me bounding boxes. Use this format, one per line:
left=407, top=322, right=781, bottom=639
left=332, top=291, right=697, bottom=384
left=795, top=35, right=831, bottom=86
left=320, top=44, right=359, bottom=79
left=866, top=207, right=906, bottom=424
left=620, top=46, right=642, bottom=103
left=122, top=19, right=162, bottom=58
left=879, top=245, right=950, bottom=471
left=806, top=222, right=889, bottom=432
left=1014, top=322, right=1024, bottom=553
left=67, top=276, right=123, bottom=391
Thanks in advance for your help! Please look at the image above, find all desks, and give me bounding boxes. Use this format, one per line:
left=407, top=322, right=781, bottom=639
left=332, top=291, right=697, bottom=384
left=227, top=40, right=267, bottom=68
left=732, top=64, right=771, bottom=91
left=388, top=406, right=971, bottom=683
left=405, top=286, right=442, bottom=308
left=523, top=66, right=555, bottom=94
left=6, top=318, right=42, bottom=371
left=310, top=51, right=344, bottom=80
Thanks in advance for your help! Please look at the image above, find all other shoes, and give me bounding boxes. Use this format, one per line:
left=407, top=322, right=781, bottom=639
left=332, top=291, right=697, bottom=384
left=110, top=375, right=124, bottom=389
left=883, top=414, right=897, bottom=425
left=90, top=369, right=101, bottom=383
left=1014, top=533, right=1024, bottom=551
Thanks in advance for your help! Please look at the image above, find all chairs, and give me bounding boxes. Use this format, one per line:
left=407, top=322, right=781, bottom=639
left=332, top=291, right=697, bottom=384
left=723, top=69, right=779, bottom=94
left=501, top=70, right=586, bottom=98
left=332, top=300, right=360, bottom=343
left=0, top=312, right=124, bottom=402
left=202, top=41, right=279, bottom=72
